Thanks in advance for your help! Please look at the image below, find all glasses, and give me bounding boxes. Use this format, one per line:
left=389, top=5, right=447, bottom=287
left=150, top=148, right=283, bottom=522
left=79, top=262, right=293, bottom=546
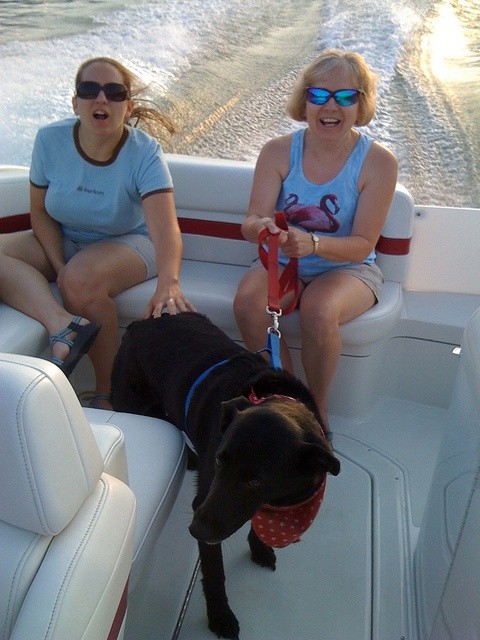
left=73, top=80, right=129, bottom=102
left=300, top=84, right=360, bottom=107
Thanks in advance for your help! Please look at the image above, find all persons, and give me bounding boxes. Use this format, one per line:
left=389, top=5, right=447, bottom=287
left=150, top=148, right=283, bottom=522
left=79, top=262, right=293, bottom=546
left=231, top=49, right=401, bottom=448
left=2, top=56, right=199, bottom=409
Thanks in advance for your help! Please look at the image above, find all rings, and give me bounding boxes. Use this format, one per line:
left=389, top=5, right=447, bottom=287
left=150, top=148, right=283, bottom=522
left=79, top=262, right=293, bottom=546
left=167, top=297, right=173, bottom=305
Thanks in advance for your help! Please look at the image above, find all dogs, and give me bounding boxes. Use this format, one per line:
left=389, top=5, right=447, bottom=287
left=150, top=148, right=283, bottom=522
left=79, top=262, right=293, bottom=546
left=110, top=312, right=340, bottom=640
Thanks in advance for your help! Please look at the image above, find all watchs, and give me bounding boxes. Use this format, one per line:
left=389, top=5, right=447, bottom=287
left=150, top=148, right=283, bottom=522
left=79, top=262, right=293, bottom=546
left=310, top=231, right=319, bottom=257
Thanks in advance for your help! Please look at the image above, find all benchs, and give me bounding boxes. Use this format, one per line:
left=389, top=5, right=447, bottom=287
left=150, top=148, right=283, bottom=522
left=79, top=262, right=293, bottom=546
left=1, top=155, right=416, bottom=346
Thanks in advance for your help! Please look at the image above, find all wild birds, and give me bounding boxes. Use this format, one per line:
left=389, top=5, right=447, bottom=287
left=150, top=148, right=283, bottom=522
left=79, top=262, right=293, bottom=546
left=286, top=194, right=340, bottom=233
left=282, top=193, right=304, bottom=222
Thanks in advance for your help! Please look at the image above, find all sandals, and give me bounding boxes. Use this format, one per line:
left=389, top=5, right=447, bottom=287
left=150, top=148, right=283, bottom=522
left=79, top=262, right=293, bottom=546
left=49, top=315, right=101, bottom=375
left=89, top=394, right=114, bottom=409
